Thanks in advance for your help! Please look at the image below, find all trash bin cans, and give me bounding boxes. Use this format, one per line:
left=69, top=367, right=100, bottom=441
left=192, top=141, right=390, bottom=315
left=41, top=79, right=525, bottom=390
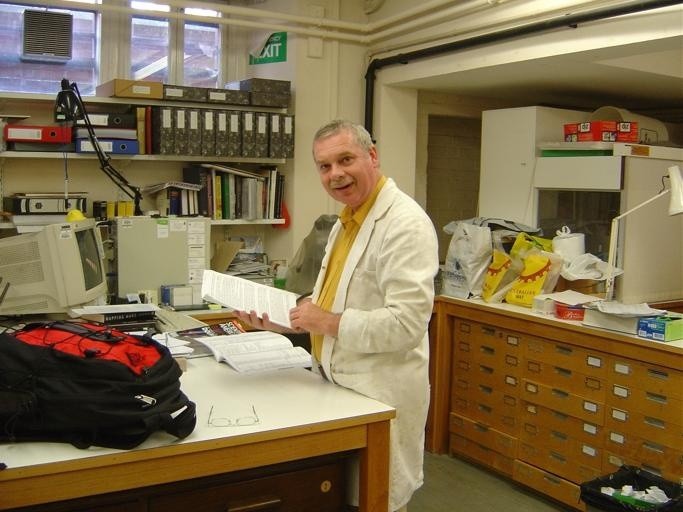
left=581, top=466, right=682, bottom=512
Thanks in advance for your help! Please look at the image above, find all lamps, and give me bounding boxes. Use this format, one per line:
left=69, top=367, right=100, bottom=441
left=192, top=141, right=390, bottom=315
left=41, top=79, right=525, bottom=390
left=55, top=78, right=143, bottom=216
left=606, top=165, right=683, bottom=303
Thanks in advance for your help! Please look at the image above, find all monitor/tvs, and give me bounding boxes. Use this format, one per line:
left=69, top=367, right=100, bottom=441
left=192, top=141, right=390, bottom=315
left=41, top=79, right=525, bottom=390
left=0, top=218, right=107, bottom=321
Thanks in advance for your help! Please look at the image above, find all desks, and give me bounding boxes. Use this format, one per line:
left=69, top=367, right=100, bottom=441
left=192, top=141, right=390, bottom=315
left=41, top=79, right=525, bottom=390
left=0, top=308, right=397, bottom=511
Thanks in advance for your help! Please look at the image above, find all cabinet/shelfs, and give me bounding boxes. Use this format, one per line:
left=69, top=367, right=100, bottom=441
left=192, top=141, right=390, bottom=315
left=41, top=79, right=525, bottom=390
left=424, top=294, right=683, bottom=512
left=0, top=91, right=290, bottom=228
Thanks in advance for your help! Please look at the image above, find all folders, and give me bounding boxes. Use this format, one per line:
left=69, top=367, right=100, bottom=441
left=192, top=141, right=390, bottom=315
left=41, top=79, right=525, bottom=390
left=4, top=196, right=86, bottom=214
left=239, top=78, right=291, bottom=106
left=3, top=124, right=71, bottom=144
left=75, top=113, right=139, bottom=154
left=163, top=83, right=249, bottom=105
left=152, top=107, right=294, bottom=158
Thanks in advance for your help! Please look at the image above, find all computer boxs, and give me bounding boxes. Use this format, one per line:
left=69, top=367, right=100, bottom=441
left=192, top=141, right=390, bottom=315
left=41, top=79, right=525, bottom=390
left=112, top=217, right=211, bottom=298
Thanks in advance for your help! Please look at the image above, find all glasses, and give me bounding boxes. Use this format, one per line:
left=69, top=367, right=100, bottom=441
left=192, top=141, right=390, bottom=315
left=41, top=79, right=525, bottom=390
left=206, top=404, right=259, bottom=429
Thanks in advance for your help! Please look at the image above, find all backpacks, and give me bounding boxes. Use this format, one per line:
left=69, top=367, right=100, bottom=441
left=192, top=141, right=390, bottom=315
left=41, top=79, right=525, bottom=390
left=0, top=317, right=196, bottom=454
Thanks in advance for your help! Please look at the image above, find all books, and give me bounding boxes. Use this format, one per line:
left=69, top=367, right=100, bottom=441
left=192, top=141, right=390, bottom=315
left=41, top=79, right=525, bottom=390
left=2, top=105, right=296, bottom=227
left=193, top=328, right=315, bottom=377
left=76, top=304, right=163, bottom=334
left=160, top=75, right=291, bottom=108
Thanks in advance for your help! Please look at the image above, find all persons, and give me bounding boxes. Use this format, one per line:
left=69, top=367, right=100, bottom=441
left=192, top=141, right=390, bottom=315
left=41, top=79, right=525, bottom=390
left=233, top=116, right=439, bottom=511
